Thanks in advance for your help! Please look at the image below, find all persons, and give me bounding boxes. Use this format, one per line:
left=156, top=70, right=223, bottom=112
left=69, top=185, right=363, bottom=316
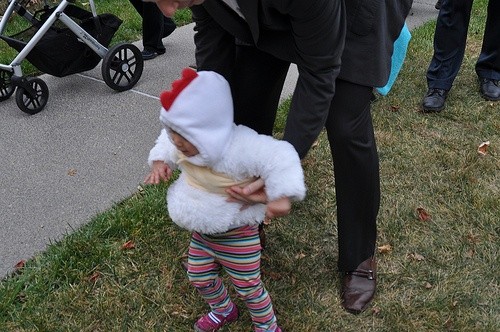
left=146, top=0, right=415, bottom=332
left=424, top=0, right=499, bottom=110
left=130, top=0, right=177, bottom=61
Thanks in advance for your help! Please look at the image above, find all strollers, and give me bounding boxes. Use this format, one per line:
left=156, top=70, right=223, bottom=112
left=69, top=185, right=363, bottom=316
left=0, top=0, right=144, bottom=114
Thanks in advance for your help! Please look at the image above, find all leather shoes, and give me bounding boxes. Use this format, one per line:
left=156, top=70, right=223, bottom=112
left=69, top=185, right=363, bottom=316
left=423, top=88, right=448, bottom=111
left=343, top=250, right=377, bottom=314
left=480, top=79, right=500, bottom=101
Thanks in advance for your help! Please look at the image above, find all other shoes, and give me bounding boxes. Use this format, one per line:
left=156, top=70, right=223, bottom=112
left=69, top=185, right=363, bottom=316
left=141, top=48, right=166, bottom=60
left=195, top=303, right=238, bottom=332
